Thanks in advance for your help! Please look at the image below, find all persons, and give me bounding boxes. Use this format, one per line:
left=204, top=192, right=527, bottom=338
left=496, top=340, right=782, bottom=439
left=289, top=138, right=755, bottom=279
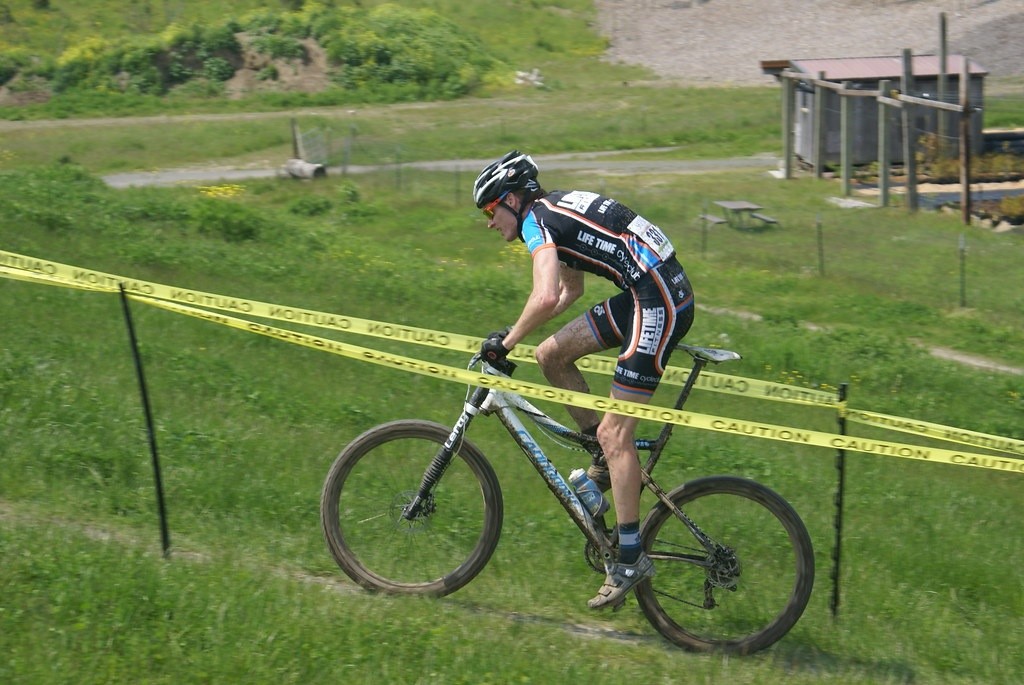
left=472, top=149, right=695, bottom=610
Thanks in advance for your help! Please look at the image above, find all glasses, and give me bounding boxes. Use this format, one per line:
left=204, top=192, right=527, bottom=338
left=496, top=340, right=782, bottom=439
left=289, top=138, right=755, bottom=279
left=483, top=188, right=511, bottom=221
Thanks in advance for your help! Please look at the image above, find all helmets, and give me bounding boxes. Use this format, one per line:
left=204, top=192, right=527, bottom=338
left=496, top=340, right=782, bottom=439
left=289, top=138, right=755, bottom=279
left=472, top=149, right=538, bottom=209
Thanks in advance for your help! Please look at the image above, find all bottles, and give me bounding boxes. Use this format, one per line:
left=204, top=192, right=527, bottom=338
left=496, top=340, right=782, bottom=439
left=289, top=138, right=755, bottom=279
left=567, top=466, right=611, bottom=519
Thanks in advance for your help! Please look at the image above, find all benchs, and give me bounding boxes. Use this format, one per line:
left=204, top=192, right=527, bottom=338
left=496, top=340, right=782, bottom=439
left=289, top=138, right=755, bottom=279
left=699, top=214, right=729, bottom=224
left=752, top=213, right=781, bottom=231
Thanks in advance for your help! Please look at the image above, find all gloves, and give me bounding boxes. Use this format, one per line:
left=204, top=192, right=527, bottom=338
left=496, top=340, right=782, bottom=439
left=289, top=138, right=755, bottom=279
left=480, top=337, right=510, bottom=364
left=487, top=326, right=511, bottom=340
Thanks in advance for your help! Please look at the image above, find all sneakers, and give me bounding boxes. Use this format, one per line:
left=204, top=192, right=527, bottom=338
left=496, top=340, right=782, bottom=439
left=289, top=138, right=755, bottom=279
left=587, top=550, right=656, bottom=610
left=586, top=462, right=611, bottom=493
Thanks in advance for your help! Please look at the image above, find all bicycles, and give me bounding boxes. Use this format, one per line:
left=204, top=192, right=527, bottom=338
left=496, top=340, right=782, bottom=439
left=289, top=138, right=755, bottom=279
left=320, top=324, right=816, bottom=658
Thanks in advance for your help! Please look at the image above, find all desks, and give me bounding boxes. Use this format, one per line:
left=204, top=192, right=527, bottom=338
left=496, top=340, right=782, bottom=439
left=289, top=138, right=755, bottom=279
left=713, top=199, right=764, bottom=230
